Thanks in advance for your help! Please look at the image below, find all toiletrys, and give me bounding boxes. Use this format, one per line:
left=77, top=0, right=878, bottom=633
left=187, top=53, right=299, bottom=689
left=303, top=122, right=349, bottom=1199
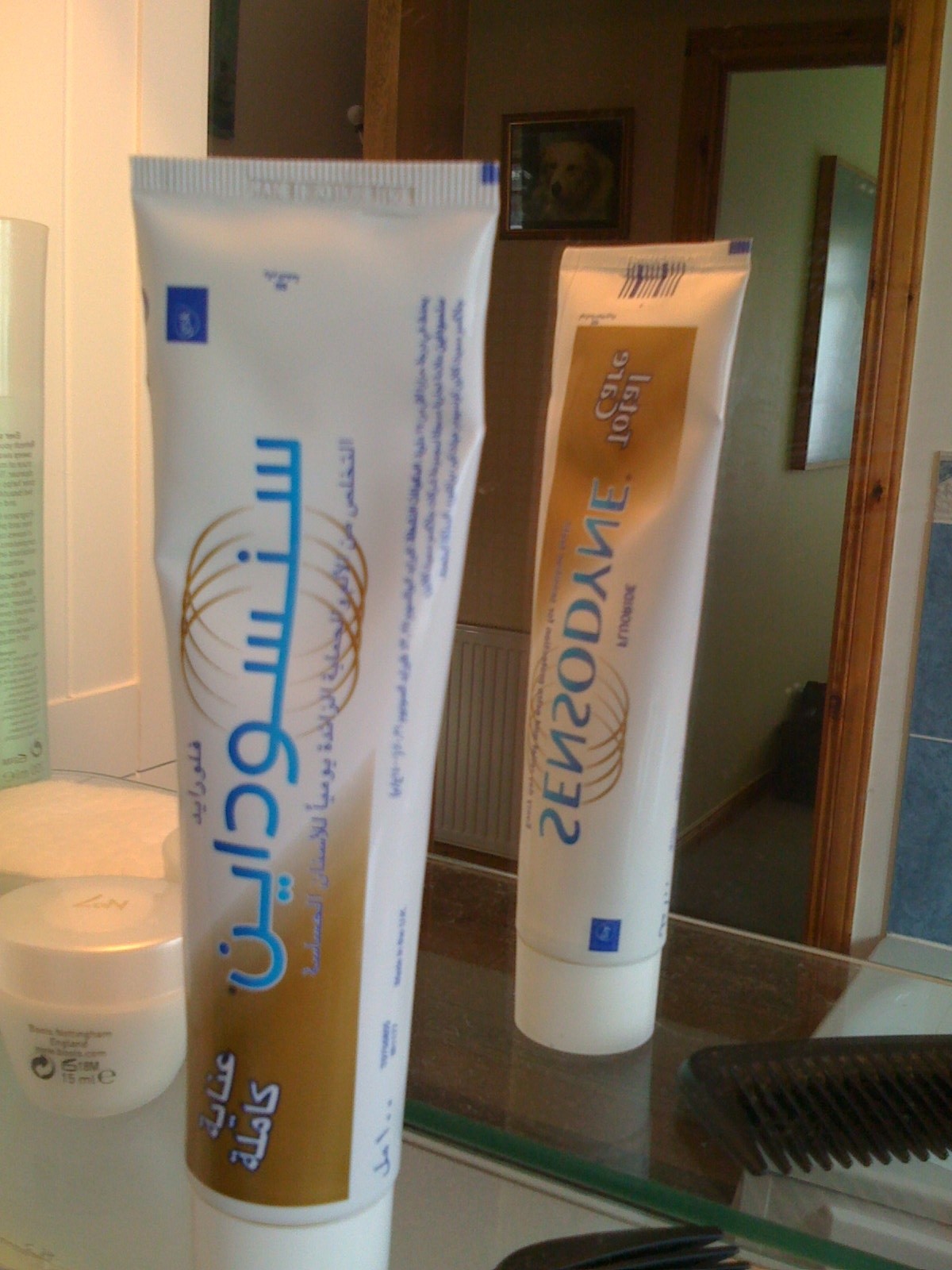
left=120, top=152, right=519, bottom=1254
left=510, top=233, right=762, bottom=1062
left=0, top=877, right=189, bottom=1123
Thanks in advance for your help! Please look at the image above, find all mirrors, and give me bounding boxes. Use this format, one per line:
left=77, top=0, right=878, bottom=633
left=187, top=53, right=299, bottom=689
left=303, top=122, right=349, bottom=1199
left=207, top=0, right=952, bottom=1270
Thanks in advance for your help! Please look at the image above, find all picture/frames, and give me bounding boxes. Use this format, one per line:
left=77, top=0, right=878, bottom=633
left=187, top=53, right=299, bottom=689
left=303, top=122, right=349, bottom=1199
left=792, top=154, right=879, bottom=470
left=500, top=107, right=635, bottom=241
